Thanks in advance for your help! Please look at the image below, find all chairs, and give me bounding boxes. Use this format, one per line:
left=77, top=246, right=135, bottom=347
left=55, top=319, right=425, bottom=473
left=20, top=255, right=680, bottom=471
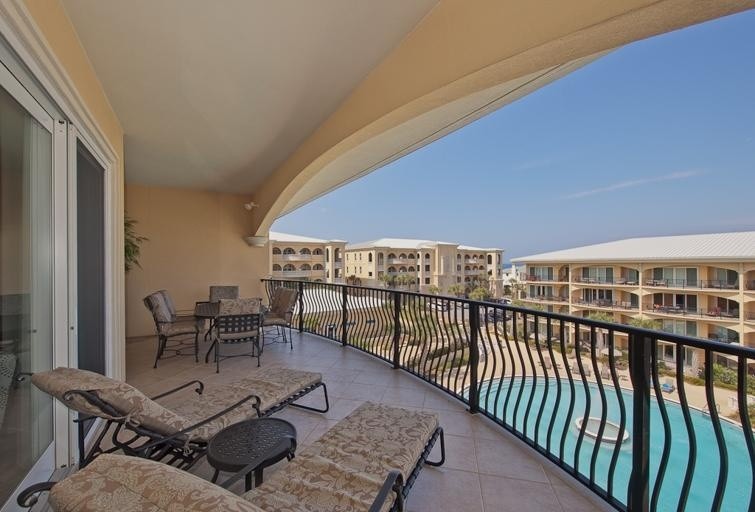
left=543, top=356, right=676, bottom=394
left=141, top=284, right=299, bottom=373
left=13, top=400, right=446, bottom=512
left=14, top=366, right=331, bottom=471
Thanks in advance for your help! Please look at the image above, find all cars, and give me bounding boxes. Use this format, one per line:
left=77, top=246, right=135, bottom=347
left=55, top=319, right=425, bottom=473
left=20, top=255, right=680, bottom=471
left=427, top=295, right=512, bottom=321
